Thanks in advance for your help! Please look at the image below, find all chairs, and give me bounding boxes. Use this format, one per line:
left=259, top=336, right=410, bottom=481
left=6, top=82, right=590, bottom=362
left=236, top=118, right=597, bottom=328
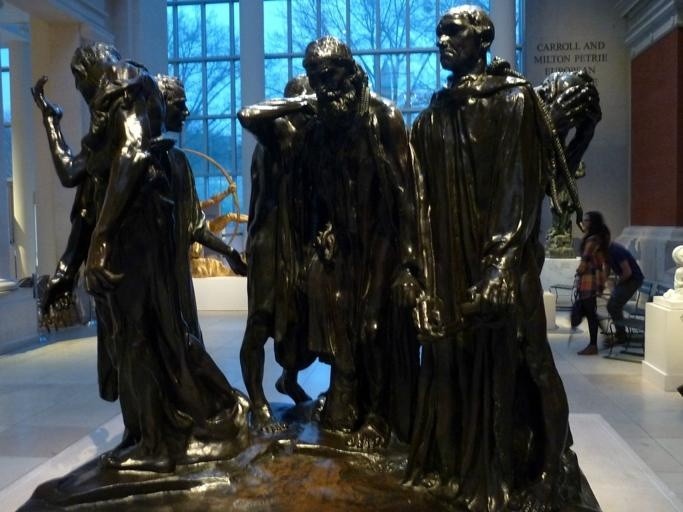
left=548, top=274, right=668, bottom=358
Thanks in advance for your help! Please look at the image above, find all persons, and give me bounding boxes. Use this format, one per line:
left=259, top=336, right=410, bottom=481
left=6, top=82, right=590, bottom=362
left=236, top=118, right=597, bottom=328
left=604, top=226, right=646, bottom=338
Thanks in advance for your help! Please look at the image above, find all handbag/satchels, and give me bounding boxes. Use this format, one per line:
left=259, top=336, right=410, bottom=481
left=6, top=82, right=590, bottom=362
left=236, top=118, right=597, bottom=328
left=570, top=299, right=586, bottom=329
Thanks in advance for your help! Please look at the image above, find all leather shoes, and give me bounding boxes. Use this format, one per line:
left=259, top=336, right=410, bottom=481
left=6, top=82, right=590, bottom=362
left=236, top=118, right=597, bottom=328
left=576, top=343, right=598, bottom=355
